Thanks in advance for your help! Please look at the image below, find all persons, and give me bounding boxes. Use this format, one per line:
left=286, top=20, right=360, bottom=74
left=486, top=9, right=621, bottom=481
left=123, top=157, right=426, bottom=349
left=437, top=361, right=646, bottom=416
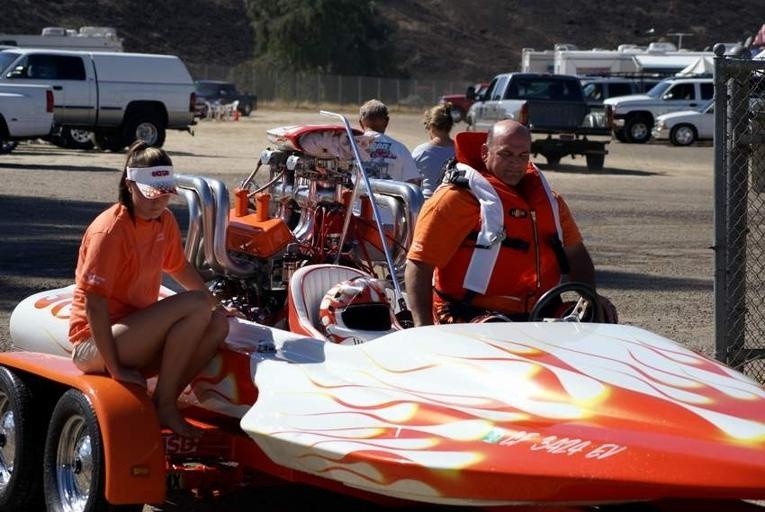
left=206, top=94, right=241, bottom=125
left=405, top=119, right=619, bottom=327
left=354, top=99, right=424, bottom=205
left=69, top=139, right=229, bottom=438
left=413, top=104, right=456, bottom=202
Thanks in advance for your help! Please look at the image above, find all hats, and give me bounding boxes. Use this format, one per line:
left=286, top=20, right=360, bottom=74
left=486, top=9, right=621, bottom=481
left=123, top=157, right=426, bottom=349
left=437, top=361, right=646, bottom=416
left=127, top=166, right=181, bottom=200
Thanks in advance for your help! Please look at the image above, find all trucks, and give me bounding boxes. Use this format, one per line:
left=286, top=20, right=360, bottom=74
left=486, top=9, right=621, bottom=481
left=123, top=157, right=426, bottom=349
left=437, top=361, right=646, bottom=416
left=632, top=55, right=718, bottom=79
left=0, top=49, right=198, bottom=149
left=521, top=48, right=554, bottom=75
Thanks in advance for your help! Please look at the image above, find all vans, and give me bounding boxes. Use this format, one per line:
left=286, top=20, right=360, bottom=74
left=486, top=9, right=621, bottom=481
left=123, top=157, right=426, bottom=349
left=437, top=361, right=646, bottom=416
left=581, top=80, right=659, bottom=134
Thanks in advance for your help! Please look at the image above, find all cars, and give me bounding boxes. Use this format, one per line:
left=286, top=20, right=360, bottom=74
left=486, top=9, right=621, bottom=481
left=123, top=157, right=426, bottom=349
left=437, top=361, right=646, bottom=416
left=651, top=97, right=762, bottom=146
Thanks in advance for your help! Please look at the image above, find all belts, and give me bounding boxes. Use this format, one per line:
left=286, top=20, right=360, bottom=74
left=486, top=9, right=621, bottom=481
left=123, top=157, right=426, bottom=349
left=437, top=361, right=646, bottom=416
left=436, top=291, right=530, bottom=324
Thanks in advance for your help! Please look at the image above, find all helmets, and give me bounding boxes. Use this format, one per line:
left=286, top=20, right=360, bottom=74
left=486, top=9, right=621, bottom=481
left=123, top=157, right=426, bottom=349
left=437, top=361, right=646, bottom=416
left=320, top=274, right=392, bottom=344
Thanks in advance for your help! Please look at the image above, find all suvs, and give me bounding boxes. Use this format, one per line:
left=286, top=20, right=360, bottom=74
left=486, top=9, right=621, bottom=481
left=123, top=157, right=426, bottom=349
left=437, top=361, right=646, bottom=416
left=442, top=84, right=489, bottom=123
left=603, top=78, right=731, bottom=143
left=194, top=81, right=257, bottom=117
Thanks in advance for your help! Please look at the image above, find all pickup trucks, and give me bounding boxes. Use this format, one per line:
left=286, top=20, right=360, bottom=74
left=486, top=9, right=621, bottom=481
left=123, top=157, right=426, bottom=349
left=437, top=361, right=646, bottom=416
left=466, top=73, right=612, bottom=169
left=0, top=83, right=54, bottom=151
left=554, top=47, right=651, bottom=78
